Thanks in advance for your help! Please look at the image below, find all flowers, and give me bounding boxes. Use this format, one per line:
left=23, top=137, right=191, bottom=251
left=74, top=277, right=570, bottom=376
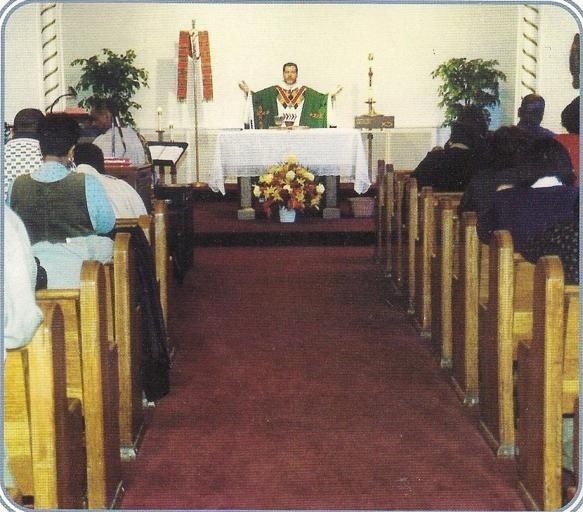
left=252, top=154, right=325, bottom=218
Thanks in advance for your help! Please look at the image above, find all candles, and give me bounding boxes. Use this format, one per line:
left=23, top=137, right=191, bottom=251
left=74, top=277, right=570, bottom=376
left=158, top=108, right=162, bottom=131
left=169, top=122, right=173, bottom=140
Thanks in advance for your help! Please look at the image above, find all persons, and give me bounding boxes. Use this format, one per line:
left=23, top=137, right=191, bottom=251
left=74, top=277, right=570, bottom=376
left=237, top=62, right=343, bottom=129
left=3, top=99, right=160, bottom=350
left=410, top=95, right=579, bottom=283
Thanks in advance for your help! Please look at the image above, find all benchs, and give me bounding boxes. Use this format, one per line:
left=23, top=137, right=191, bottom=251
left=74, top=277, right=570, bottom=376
left=3, top=200, right=172, bottom=509
left=375, top=158, right=582, bottom=511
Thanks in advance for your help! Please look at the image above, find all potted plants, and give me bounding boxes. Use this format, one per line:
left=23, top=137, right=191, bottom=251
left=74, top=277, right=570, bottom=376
left=70, top=46, right=151, bottom=169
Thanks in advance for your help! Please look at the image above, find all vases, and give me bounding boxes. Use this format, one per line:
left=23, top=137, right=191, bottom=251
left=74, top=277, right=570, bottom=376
left=348, top=196, right=375, bottom=218
left=279, top=205, right=296, bottom=222
